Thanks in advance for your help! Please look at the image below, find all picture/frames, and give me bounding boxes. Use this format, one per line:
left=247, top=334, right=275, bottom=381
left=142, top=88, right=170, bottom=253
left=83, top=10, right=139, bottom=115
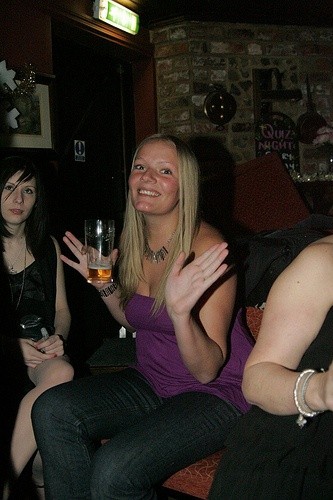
left=0, top=68, right=57, bottom=161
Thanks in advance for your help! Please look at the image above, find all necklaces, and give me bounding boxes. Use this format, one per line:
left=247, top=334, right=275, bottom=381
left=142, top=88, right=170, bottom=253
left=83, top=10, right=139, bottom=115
left=144, top=231, right=175, bottom=264
left=2, top=235, right=27, bottom=313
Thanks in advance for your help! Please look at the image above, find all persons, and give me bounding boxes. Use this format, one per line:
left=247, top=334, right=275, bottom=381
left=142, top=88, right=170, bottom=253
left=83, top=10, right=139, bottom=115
left=206, top=235, right=333, bottom=500
left=0, top=155, right=74, bottom=500
left=31, top=134, right=254, bottom=500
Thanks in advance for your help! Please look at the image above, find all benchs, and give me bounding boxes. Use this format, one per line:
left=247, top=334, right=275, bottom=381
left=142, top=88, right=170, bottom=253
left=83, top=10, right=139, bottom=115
left=99, top=307, right=263, bottom=500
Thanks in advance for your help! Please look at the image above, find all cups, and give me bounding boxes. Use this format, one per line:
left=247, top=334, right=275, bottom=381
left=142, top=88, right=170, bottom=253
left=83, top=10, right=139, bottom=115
left=85, top=219, right=114, bottom=283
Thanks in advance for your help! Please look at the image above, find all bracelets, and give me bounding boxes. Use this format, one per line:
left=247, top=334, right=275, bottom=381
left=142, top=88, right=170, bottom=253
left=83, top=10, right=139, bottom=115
left=98, top=282, right=119, bottom=298
left=293, top=368, right=327, bottom=426
